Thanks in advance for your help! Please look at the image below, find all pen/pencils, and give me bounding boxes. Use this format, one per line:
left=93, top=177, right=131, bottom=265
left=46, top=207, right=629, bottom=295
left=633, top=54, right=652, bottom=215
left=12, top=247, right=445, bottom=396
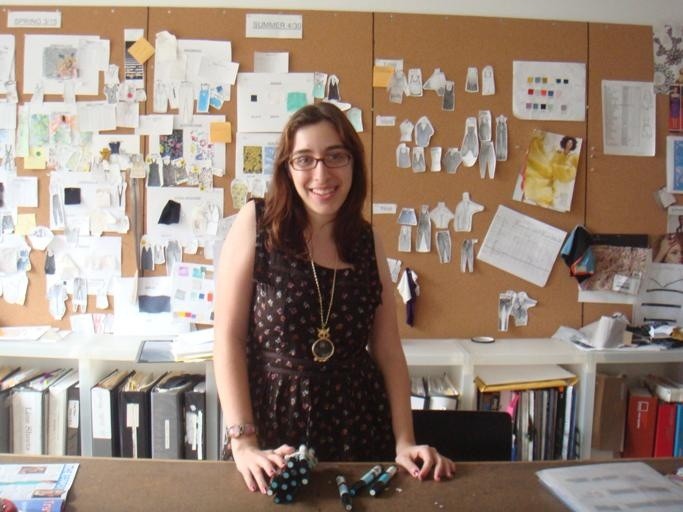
left=266, top=443, right=319, bottom=504
left=337, top=464, right=399, bottom=511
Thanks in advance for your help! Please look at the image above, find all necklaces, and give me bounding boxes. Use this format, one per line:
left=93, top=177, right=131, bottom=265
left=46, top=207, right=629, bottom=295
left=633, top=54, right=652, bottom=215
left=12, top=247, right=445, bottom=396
left=288, top=218, right=343, bottom=363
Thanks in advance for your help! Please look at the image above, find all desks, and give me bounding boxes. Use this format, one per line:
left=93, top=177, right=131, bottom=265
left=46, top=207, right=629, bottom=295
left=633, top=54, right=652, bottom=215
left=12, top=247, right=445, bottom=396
left=1, top=453, right=683, bottom=511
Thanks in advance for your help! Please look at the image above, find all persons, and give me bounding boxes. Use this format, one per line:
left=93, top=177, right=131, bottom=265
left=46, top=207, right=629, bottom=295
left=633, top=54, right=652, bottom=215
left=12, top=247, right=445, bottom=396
left=210, top=101, right=458, bottom=499
left=652, top=232, right=683, bottom=267
left=515, top=135, right=578, bottom=210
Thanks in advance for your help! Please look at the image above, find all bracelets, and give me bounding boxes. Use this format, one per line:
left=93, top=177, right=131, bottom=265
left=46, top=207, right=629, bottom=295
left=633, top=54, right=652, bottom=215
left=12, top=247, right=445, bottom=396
left=222, top=424, right=260, bottom=440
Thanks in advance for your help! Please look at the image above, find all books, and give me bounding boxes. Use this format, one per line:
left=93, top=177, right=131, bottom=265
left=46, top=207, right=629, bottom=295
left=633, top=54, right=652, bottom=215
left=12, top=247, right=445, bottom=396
left=477, top=382, right=580, bottom=464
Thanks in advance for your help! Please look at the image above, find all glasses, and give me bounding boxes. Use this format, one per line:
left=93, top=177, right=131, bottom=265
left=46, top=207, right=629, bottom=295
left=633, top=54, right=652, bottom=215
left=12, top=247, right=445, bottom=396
left=289, top=151, right=352, bottom=172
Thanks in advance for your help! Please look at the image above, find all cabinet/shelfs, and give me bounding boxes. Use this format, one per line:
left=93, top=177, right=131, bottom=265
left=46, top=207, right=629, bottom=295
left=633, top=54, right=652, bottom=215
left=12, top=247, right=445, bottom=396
left=0, top=327, right=683, bottom=458
